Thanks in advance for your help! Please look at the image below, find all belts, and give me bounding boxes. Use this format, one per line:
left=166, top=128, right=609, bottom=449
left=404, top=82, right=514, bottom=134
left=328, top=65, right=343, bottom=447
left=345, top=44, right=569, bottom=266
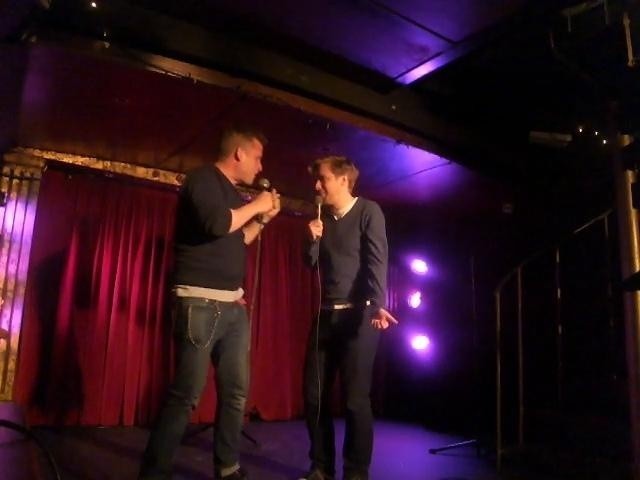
left=321, top=300, right=364, bottom=313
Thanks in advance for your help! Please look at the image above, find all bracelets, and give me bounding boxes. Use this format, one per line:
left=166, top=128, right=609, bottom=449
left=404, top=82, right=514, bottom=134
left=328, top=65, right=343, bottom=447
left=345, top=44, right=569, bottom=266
left=257, top=217, right=267, bottom=226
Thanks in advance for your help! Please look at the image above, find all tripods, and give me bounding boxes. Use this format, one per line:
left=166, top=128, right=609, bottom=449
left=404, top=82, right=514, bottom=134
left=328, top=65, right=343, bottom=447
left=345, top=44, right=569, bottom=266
left=428, top=262, right=513, bottom=465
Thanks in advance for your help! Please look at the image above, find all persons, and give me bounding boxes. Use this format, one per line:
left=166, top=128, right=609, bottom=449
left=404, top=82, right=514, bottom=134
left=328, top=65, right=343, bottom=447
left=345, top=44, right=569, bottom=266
left=298, top=156, right=399, bottom=480
left=136, top=129, right=281, bottom=479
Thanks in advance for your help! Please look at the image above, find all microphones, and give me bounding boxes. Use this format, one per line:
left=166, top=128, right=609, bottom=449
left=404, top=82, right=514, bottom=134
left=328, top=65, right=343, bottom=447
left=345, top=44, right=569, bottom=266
left=314, top=196, right=322, bottom=241
left=256, top=178, right=270, bottom=222
